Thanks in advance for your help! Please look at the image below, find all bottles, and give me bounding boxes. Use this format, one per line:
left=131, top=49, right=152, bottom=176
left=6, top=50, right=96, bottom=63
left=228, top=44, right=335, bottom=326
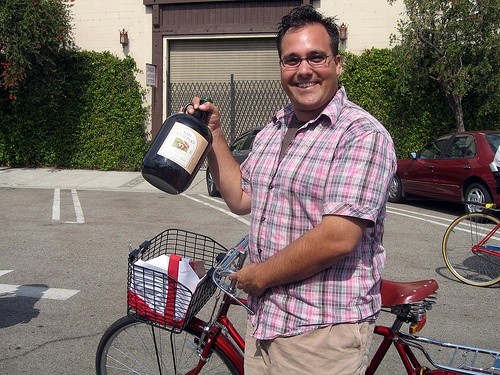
left=142, top=97, right=214, bottom=194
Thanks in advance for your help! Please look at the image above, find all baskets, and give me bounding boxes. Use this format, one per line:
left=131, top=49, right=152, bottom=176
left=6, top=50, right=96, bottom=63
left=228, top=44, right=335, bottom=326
left=127, top=229, right=229, bottom=334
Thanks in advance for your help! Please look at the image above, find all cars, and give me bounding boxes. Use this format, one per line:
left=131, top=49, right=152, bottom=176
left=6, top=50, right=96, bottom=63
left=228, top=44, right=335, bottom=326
left=387, top=131, right=500, bottom=223
left=205, top=127, right=264, bottom=197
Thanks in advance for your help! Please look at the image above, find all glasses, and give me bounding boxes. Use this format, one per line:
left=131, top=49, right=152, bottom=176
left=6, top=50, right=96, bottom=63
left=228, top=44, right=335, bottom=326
left=280, top=53, right=336, bottom=68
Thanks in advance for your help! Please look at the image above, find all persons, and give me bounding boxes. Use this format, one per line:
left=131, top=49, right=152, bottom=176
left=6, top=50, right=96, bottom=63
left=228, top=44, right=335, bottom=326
left=177, top=6, right=397, bottom=375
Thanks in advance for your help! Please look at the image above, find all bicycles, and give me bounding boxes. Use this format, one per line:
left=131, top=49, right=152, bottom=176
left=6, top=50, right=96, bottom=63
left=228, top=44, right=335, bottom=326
left=442, top=199, right=500, bottom=286
left=95, top=229, right=500, bottom=375
